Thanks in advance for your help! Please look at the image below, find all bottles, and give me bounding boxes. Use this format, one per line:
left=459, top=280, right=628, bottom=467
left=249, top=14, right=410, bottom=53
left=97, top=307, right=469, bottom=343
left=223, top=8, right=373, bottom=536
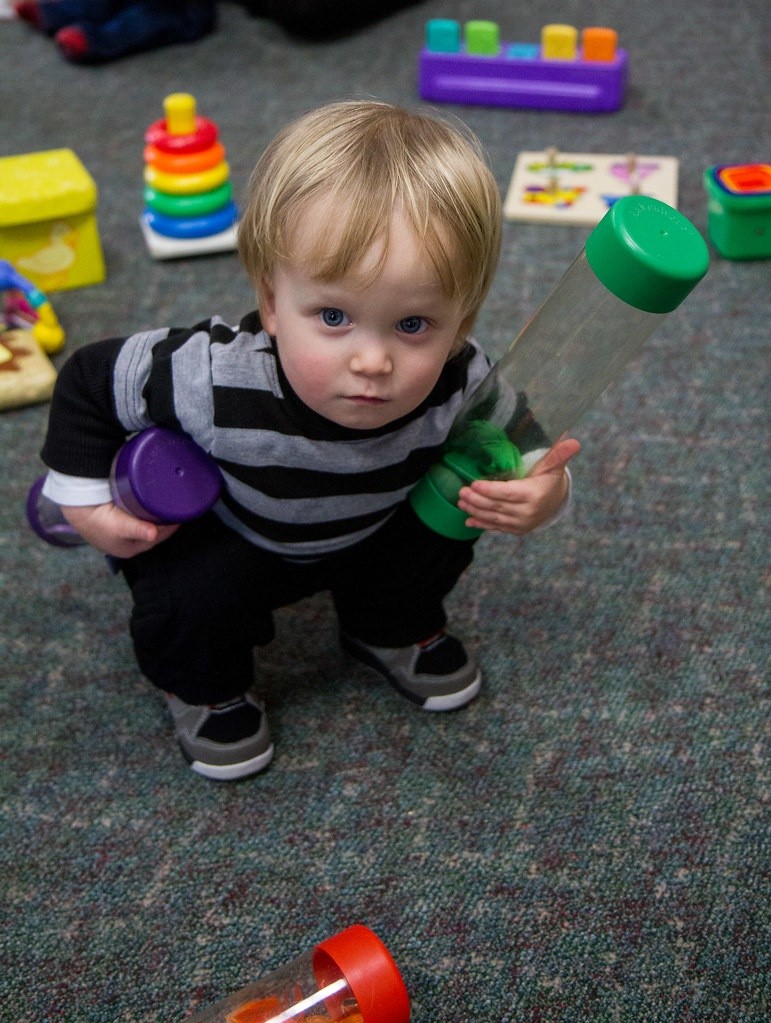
left=408, top=194, right=710, bottom=539
left=184, top=924, right=409, bottom=1023
left=25, top=426, right=220, bottom=544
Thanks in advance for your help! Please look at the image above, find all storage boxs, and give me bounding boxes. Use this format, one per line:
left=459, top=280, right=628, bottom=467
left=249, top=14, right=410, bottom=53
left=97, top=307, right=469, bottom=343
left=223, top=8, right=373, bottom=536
left=0, top=148, right=105, bottom=296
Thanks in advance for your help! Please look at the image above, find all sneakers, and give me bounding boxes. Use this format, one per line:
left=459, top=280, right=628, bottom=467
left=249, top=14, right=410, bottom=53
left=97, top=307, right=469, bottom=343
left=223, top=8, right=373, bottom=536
left=163, top=688, right=277, bottom=778
left=342, top=624, right=482, bottom=710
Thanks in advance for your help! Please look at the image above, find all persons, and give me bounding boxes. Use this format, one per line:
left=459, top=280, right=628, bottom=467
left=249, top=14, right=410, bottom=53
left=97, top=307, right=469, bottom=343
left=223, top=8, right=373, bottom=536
left=40, top=100, right=584, bottom=781
